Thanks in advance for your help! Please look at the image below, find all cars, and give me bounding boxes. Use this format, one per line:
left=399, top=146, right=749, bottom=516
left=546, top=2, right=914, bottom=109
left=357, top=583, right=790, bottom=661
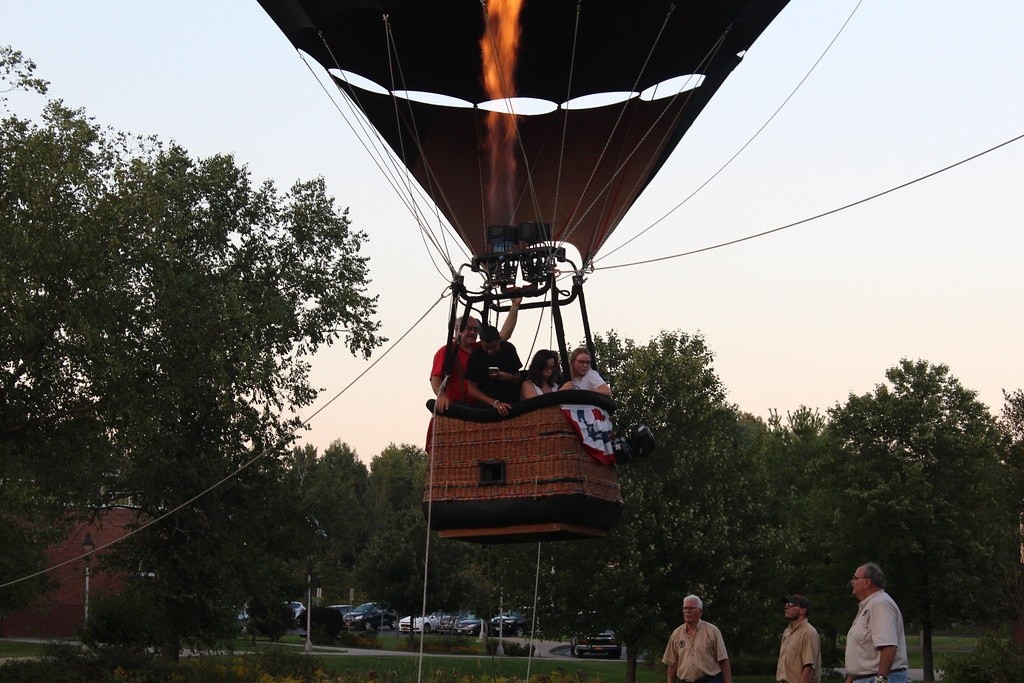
left=455, top=616, right=488, bottom=636
left=326, top=604, right=357, bottom=617
left=437, top=616, right=461, bottom=635
left=398, top=611, right=450, bottom=635
left=287, top=602, right=307, bottom=625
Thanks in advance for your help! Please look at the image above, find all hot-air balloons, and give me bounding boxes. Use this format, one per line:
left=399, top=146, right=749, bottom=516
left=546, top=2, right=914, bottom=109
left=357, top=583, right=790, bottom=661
left=256, top=0, right=794, bottom=544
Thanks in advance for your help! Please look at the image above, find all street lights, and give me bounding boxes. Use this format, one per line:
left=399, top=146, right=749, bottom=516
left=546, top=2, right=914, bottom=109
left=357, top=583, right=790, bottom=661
left=81, top=530, right=94, bottom=654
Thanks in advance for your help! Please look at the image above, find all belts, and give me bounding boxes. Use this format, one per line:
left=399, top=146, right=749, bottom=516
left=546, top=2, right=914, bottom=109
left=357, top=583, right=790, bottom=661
left=852, top=667, right=907, bottom=681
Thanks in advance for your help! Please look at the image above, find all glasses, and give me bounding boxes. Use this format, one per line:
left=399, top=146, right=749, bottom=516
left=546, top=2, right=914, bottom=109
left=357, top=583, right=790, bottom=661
left=574, top=359, right=591, bottom=365
left=783, top=602, right=805, bottom=608
left=683, top=606, right=702, bottom=611
left=852, top=576, right=867, bottom=581
left=544, top=365, right=557, bottom=372
left=457, top=324, right=480, bottom=333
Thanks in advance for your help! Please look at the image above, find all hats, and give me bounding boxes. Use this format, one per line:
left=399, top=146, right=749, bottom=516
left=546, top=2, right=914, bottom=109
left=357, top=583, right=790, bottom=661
left=782, top=594, right=812, bottom=614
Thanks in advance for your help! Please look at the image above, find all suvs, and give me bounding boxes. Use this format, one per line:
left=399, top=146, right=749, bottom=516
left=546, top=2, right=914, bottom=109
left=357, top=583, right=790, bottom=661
left=341, top=602, right=399, bottom=631
left=491, top=610, right=540, bottom=638
left=569, top=611, right=622, bottom=659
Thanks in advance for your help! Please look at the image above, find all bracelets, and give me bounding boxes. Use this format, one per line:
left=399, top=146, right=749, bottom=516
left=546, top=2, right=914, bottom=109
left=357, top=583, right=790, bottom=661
left=875, top=674, right=888, bottom=681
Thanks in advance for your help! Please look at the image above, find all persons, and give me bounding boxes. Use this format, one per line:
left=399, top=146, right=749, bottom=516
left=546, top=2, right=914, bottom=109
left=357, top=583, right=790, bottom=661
left=774, top=594, right=822, bottom=682
left=462, top=325, right=523, bottom=415
left=661, top=594, right=730, bottom=683
left=844, top=562, right=908, bottom=683
left=520, top=348, right=575, bottom=399
left=425, top=295, right=522, bottom=454
left=561, top=348, right=612, bottom=396
